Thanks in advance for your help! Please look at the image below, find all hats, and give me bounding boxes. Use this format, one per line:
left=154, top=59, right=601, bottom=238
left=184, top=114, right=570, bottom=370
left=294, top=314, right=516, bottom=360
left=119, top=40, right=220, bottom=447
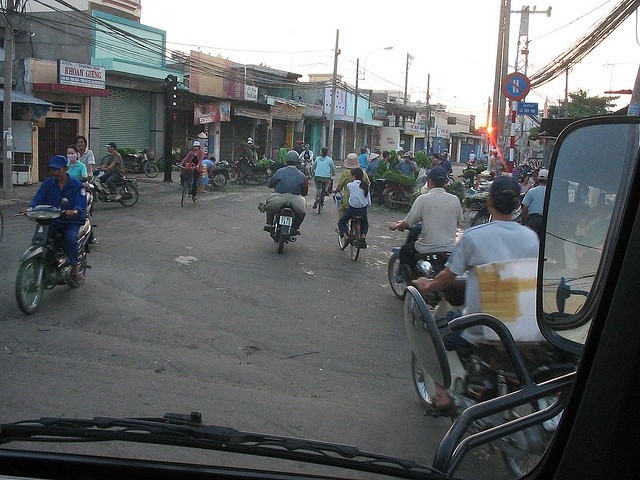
left=396, top=147, right=403, bottom=152
left=105, top=141, right=116, bottom=148
left=429, top=167, right=447, bottom=181
left=441, top=152, right=448, bottom=157
left=491, top=176, right=521, bottom=204
left=361, top=147, right=369, bottom=152
left=248, top=137, right=253, bottom=144
left=368, top=153, right=380, bottom=163
left=343, top=153, right=361, bottom=168
left=48, top=155, right=67, bottom=168
left=403, top=152, right=409, bottom=157
left=193, top=141, right=200, bottom=147
left=305, top=144, right=309, bottom=148
left=537, top=169, right=549, bottom=180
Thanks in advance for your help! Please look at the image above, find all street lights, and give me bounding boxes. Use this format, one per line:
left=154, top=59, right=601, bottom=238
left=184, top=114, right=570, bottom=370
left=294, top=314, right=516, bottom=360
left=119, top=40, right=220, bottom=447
left=353, top=45, right=393, bottom=152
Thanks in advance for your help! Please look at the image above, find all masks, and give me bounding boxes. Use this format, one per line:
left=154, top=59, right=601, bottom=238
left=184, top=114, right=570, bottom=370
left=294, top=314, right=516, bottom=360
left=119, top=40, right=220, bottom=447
left=67, top=153, right=78, bottom=161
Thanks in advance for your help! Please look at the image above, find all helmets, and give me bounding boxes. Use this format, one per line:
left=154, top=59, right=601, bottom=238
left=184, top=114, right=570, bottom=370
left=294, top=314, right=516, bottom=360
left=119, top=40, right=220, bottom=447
left=284, top=150, right=299, bottom=162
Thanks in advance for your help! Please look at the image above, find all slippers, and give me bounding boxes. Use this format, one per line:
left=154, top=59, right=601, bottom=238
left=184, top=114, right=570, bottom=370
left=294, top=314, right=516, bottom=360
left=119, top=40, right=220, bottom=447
left=73, top=273, right=85, bottom=285
left=433, top=394, right=454, bottom=411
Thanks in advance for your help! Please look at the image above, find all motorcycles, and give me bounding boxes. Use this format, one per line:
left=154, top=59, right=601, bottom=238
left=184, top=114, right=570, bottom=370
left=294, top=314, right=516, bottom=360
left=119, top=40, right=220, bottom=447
left=91, top=173, right=140, bottom=208
left=123, top=145, right=160, bottom=178
left=267, top=187, right=305, bottom=255
left=12, top=203, right=98, bottom=314
left=202, top=162, right=228, bottom=189
left=407, top=282, right=562, bottom=475
left=385, top=224, right=466, bottom=300
left=469, top=193, right=526, bottom=230
left=228, top=159, right=269, bottom=186
left=82, top=185, right=94, bottom=216
left=382, top=181, right=421, bottom=210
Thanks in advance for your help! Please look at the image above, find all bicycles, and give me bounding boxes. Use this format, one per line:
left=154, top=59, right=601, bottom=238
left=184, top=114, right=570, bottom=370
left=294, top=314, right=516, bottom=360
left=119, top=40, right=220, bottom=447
left=177, top=166, right=203, bottom=209
left=300, top=159, right=312, bottom=184
left=310, top=173, right=333, bottom=217
left=333, top=189, right=366, bottom=261
left=448, top=174, right=456, bottom=187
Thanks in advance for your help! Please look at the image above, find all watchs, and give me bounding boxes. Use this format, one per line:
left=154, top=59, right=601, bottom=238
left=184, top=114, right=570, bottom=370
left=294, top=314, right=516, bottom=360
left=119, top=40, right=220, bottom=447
left=74, top=210, right=78, bottom=216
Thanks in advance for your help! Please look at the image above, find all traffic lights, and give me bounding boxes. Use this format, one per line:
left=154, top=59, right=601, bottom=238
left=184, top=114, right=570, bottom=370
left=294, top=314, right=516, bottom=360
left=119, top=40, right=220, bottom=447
left=172, top=113, right=178, bottom=124
left=164, top=74, right=178, bottom=110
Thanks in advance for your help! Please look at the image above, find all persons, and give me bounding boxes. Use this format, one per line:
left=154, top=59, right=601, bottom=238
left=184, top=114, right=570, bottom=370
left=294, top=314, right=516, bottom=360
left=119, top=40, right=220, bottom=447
left=410, top=176, right=547, bottom=408
left=272, top=143, right=288, bottom=167
left=521, top=168, right=549, bottom=237
left=263, top=150, right=309, bottom=235
left=332, top=152, right=371, bottom=235
left=68, top=135, right=96, bottom=172
left=298, top=144, right=314, bottom=179
left=358, top=145, right=453, bottom=206
left=66, top=144, right=88, bottom=186
left=337, top=168, right=371, bottom=248
left=180, top=140, right=204, bottom=198
left=389, top=166, right=464, bottom=284
left=19, top=155, right=88, bottom=285
left=197, top=157, right=216, bottom=193
left=100, top=142, right=125, bottom=200
